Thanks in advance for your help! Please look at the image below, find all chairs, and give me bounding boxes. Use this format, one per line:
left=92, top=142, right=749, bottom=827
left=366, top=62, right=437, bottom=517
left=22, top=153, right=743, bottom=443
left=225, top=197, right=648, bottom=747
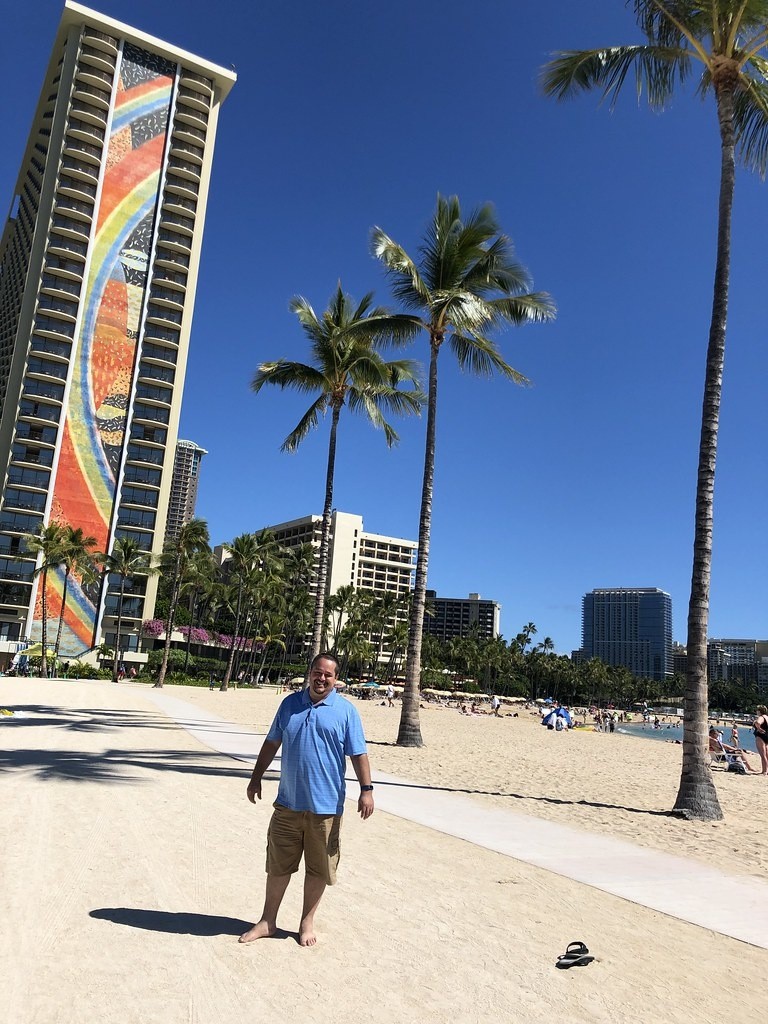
left=709, top=737, right=746, bottom=771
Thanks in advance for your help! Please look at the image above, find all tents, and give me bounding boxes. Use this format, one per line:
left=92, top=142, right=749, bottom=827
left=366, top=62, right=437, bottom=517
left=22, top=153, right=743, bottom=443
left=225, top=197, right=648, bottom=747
left=541, top=707, right=574, bottom=729
left=16, top=643, right=57, bottom=678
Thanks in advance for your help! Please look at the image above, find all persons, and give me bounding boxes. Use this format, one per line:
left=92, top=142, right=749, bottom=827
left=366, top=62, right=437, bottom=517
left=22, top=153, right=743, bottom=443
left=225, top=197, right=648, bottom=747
left=634, top=711, right=683, bottom=744
left=238, top=652, right=374, bottom=947
left=64, top=660, right=70, bottom=671
left=118, top=664, right=125, bottom=681
left=349, top=687, right=597, bottom=722
left=129, top=666, right=136, bottom=678
left=708, top=715, right=757, bottom=772
left=593, top=709, right=632, bottom=733
left=752, top=704, right=768, bottom=776
left=8, top=658, right=13, bottom=669
left=387, top=681, right=395, bottom=707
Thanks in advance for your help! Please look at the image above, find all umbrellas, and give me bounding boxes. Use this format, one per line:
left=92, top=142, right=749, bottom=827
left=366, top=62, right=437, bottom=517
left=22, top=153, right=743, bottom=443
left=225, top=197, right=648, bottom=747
left=647, top=708, right=653, bottom=713
left=334, top=680, right=527, bottom=702
left=536, top=697, right=544, bottom=703
left=545, top=698, right=553, bottom=703
left=290, top=676, right=305, bottom=688
left=607, top=704, right=614, bottom=708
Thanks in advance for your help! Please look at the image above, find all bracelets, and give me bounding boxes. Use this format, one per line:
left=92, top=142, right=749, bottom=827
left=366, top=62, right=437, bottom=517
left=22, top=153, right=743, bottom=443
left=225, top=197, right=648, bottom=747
left=361, top=785, right=373, bottom=790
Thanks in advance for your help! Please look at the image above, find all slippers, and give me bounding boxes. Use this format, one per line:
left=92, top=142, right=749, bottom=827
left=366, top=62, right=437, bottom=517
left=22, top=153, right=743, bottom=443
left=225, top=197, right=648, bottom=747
left=557, top=940, right=595, bottom=968
